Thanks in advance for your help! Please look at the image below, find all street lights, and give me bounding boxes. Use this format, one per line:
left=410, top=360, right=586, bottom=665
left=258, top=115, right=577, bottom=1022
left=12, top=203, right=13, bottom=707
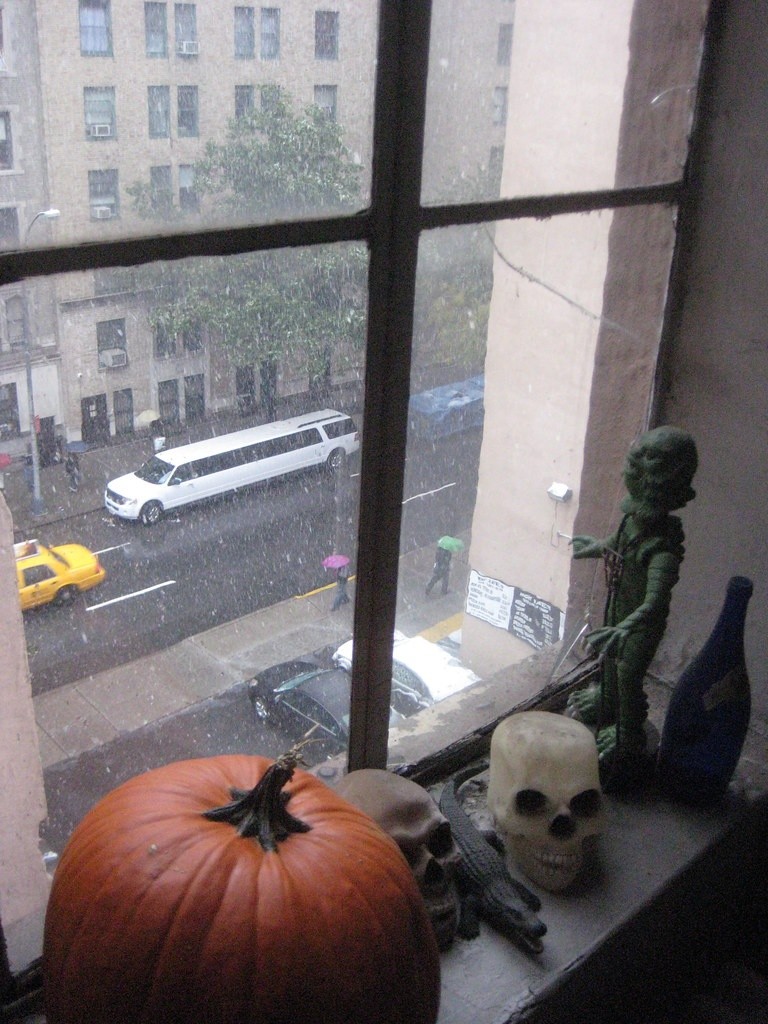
left=19, top=207, right=62, bottom=516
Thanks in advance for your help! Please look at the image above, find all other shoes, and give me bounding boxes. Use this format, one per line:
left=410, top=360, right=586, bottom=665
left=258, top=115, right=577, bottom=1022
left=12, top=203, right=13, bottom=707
left=426, top=589, right=451, bottom=595
left=330, top=599, right=349, bottom=611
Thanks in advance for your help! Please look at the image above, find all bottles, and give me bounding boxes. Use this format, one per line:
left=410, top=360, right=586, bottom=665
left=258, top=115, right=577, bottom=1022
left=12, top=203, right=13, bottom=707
left=652, top=573, right=754, bottom=800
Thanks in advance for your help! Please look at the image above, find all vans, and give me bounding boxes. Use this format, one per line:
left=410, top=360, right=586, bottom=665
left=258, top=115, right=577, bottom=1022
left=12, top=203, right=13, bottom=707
left=103, top=409, right=361, bottom=527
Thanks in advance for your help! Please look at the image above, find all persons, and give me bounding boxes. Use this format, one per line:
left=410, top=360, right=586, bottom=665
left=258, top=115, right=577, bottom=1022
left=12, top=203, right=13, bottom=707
left=141, top=420, right=165, bottom=467
left=329, top=564, right=350, bottom=613
left=64, top=452, right=81, bottom=494
left=425, top=547, right=452, bottom=597
left=568, top=426, right=700, bottom=784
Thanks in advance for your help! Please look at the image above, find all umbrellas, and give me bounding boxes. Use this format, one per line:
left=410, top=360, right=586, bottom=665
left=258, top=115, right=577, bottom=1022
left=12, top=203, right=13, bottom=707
left=134, top=409, right=161, bottom=427
left=62, top=440, right=90, bottom=453
left=321, top=554, right=351, bottom=569
left=437, top=536, right=465, bottom=554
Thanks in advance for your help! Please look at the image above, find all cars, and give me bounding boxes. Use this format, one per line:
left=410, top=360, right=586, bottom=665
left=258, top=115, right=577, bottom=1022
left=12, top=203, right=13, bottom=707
left=332, top=627, right=484, bottom=715
left=14, top=537, right=106, bottom=612
left=246, top=659, right=407, bottom=761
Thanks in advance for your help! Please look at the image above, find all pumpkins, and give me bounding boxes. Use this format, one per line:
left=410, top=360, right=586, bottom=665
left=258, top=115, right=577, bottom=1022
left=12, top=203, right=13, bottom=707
left=41, top=750, right=441, bottom=1023
